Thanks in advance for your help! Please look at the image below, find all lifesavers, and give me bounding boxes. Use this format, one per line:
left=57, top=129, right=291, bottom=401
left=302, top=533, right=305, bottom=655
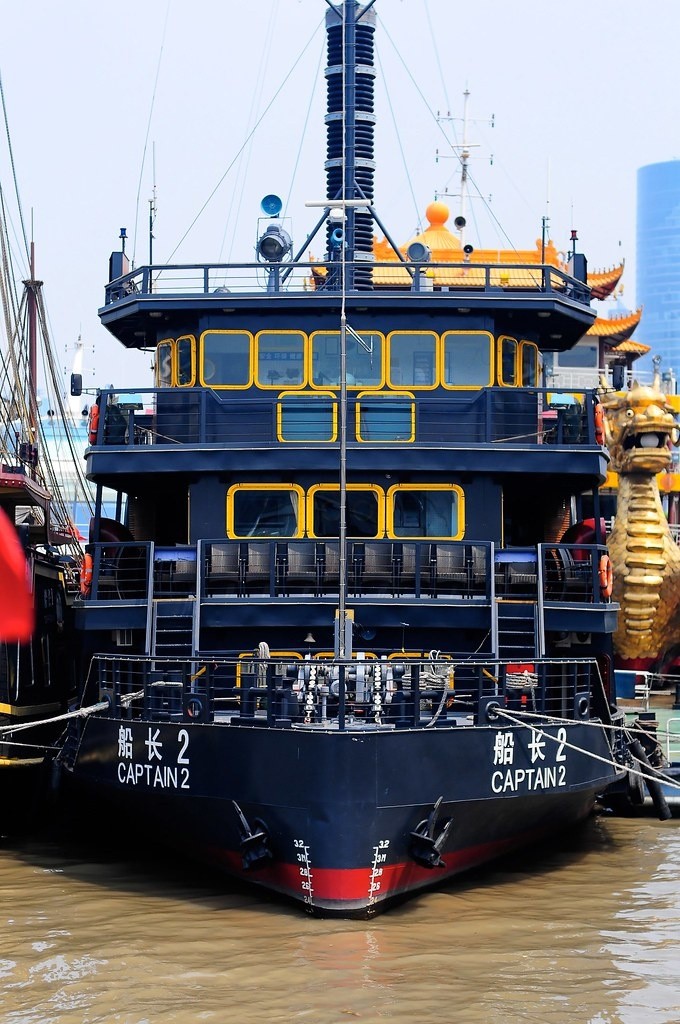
left=88, top=404, right=98, bottom=444
left=80, top=553, right=93, bottom=593
left=600, top=554, right=614, bottom=597
left=595, top=403, right=607, bottom=446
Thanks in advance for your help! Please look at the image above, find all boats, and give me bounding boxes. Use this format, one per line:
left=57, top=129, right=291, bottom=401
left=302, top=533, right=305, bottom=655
left=32, top=0, right=666, bottom=918
left=0, top=71, right=97, bottom=837
